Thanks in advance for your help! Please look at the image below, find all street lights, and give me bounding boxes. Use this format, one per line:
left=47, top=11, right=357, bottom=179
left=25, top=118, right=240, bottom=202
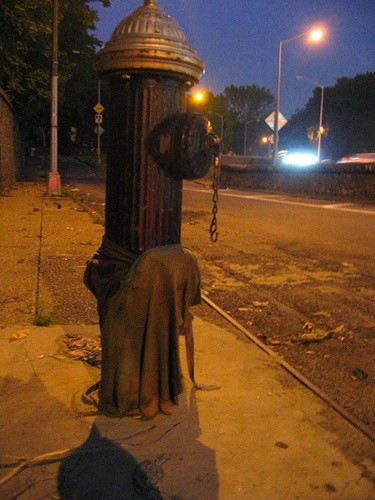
left=244, top=119, right=261, bottom=156
left=297, top=76, right=325, bottom=164
left=271, top=26, right=324, bottom=165
left=181, top=91, right=204, bottom=114
left=47, top=2, right=114, bottom=198
left=207, top=110, right=224, bottom=141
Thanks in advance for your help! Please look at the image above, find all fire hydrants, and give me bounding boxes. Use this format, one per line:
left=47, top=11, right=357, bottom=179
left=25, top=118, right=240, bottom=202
left=83, top=1, right=222, bottom=415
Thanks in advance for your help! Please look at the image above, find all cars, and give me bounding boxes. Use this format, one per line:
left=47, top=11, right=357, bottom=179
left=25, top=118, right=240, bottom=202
left=336, top=152, right=375, bottom=163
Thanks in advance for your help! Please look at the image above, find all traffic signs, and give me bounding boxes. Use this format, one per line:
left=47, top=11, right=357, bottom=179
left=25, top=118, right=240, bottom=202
left=93, top=104, right=105, bottom=114
left=95, top=115, right=103, bottom=124
left=94, top=127, right=105, bottom=137
left=265, top=111, right=288, bottom=135
